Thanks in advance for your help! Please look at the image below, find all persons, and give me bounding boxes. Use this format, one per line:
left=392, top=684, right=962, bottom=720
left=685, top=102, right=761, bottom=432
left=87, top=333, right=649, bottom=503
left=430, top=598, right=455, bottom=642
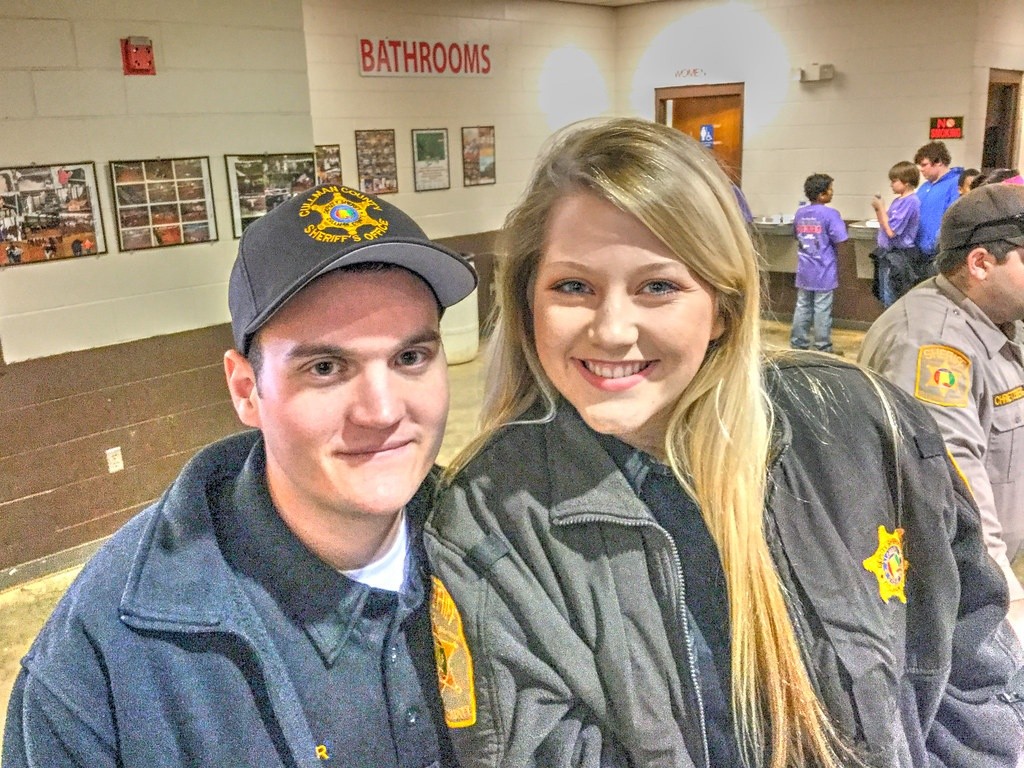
left=858, top=182, right=1024, bottom=656
left=788, top=175, right=849, bottom=355
left=871, top=141, right=1023, bottom=322
left=4, top=237, right=95, bottom=264
left=426, top=120, right=1023, bottom=767
left=1, top=184, right=453, bottom=768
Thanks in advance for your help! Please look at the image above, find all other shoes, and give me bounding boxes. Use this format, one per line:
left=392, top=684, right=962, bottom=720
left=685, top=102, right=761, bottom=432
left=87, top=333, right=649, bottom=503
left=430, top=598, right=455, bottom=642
left=826, top=350, right=845, bottom=358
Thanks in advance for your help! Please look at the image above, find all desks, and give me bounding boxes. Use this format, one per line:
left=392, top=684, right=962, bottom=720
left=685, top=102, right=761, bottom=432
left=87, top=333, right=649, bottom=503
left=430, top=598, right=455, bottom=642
left=751, top=219, right=881, bottom=241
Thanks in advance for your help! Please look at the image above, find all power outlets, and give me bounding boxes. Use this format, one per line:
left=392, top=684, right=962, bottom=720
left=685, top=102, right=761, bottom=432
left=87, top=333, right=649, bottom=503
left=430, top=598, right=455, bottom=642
left=106, top=447, right=124, bottom=475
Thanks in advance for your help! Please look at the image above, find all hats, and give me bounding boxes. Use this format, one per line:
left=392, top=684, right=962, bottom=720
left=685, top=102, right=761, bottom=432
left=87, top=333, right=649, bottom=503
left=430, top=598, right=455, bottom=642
left=229, top=183, right=477, bottom=355
left=941, top=183, right=1024, bottom=250
